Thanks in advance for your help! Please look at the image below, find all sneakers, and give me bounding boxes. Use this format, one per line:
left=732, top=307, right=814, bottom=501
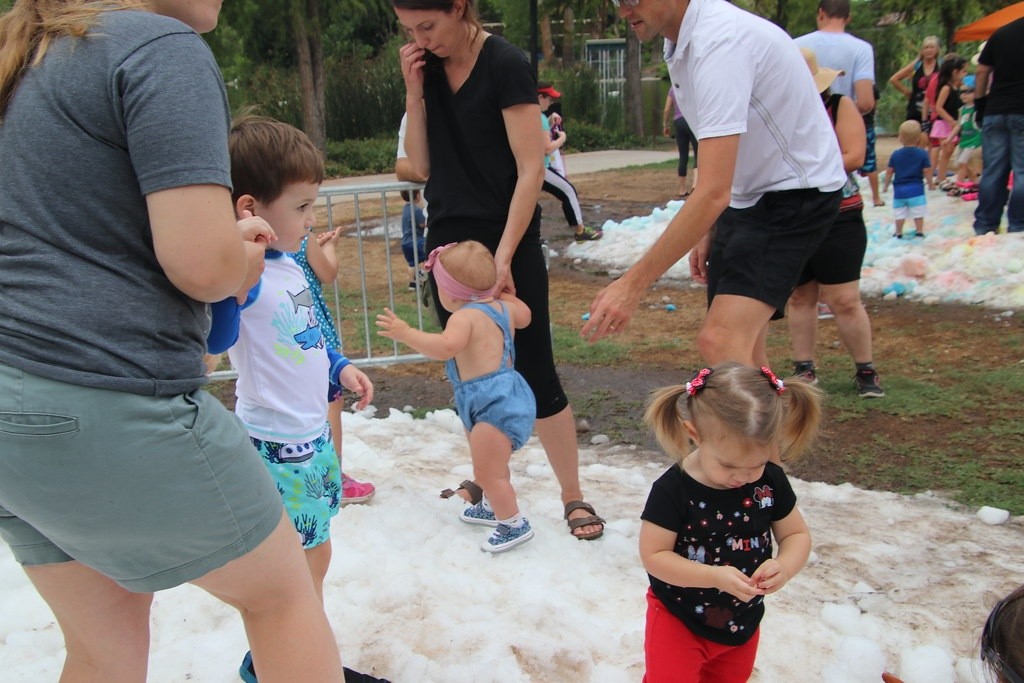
left=852, top=369, right=884, bottom=398
left=481, top=517, right=533, bottom=553
left=574, top=227, right=603, bottom=244
left=781, top=364, right=819, bottom=387
left=340, top=473, right=375, bottom=502
left=460, top=500, right=498, bottom=525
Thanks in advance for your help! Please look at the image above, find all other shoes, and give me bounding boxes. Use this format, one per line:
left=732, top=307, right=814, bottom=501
left=874, top=202, right=885, bottom=206
left=678, top=191, right=691, bottom=199
left=894, top=233, right=901, bottom=238
left=408, top=282, right=422, bottom=291
left=916, top=232, right=922, bottom=237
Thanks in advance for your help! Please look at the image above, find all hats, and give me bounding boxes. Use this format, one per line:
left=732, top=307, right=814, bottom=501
left=537, top=86, right=561, bottom=98
left=799, top=47, right=845, bottom=95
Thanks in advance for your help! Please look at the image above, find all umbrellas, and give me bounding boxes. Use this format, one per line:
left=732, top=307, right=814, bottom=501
left=954, top=0, right=1024, bottom=44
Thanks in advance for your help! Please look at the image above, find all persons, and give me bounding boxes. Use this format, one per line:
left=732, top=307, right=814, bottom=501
left=640, top=363, right=821, bottom=683
left=882, top=119, right=936, bottom=239
left=889, top=33, right=993, bottom=195
left=856, top=83, right=885, bottom=206
left=794, top=0, right=875, bottom=114
left=660, top=85, right=700, bottom=199
left=530, top=78, right=603, bottom=242
left=0, top=1, right=353, bottom=683
left=394, top=110, right=429, bottom=238
left=400, top=189, right=428, bottom=292
left=373, top=237, right=538, bottom=554
left=781, top=46, right=887, bottom=399
left=385, top=0, right=610, bottom=541
left=879, top=583, right=1024, bottom=683
left=200, top=108, right=390, bottom=683
left=973, top=14, right=1024, bottom=235
left=580, top=0, right=846, bottom=467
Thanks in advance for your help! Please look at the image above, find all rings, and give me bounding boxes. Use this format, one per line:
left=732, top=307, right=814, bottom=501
left=610, top=325, right=616, bottom=330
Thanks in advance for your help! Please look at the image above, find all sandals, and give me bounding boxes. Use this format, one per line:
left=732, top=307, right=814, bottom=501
left=564, top=501, right=606, bottom=540
left=439, top=479, right=482, bottom=505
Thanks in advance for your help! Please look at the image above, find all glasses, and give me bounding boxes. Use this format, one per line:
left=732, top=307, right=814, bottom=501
left=612, top=0, right=639, bottom=7
left=958, top=89, right=974, bottom=94
